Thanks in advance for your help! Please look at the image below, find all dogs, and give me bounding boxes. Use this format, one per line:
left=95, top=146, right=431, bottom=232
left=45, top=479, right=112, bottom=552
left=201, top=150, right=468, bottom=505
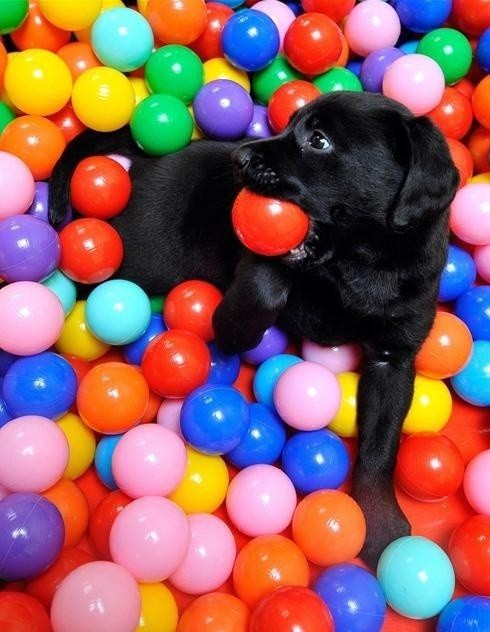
left=46, top=91, right=461, bottom=570
